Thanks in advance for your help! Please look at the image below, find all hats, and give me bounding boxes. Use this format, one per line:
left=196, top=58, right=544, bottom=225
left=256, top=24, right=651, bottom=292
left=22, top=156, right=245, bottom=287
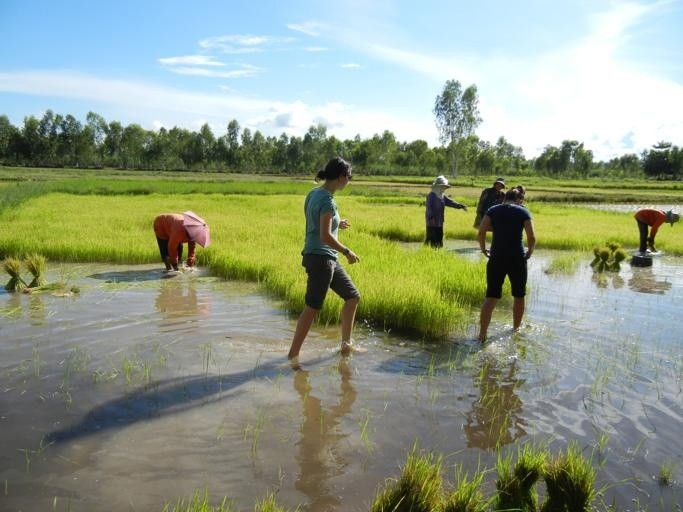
left=432, top=177, right=451, bottom=188
left=184, top=211, right=209, bottom=248
left=670, top=211, right=679, bottom=227
left=493, top=178, right=505, bottom=188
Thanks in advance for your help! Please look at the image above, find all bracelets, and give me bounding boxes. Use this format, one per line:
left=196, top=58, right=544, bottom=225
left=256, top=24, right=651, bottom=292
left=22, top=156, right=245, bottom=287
left=342, top=248, right=349, bottom=256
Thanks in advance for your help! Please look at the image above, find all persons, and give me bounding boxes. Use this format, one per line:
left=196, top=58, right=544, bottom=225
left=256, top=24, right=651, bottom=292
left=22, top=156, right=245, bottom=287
left=285, top=157, right=360, bottom=371
left=472, top=177, right=505, bottom=232
left=633, top=208, right=679, bottom=254
left=458, top=344, right=529, bottom=454
left=153, top=285, right=208, bottom=318
left=287, top=350, right=360, bottom=511
left=477, top=183, right=535, bottom=342
left=424, top=175, right=467, bottom=249
left=153, top=209, right=209, bottom=272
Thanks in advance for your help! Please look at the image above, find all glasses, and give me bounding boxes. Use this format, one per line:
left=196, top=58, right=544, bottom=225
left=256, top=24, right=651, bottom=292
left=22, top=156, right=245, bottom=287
left=346, top=174, right=352, bottom=181
